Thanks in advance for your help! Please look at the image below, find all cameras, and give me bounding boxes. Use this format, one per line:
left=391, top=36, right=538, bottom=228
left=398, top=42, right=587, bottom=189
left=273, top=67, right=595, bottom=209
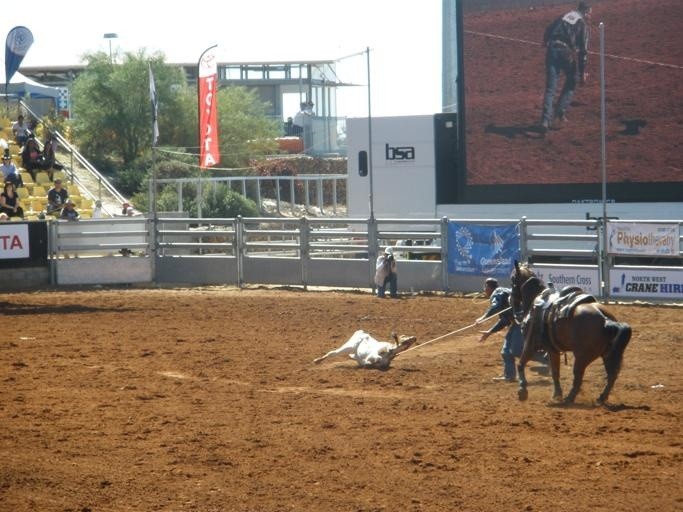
left=386, top=254, right=392, bottom=261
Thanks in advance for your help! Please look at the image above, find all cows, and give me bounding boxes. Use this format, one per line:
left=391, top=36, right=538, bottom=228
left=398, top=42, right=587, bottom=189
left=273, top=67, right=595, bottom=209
left=313, top=328, right=416, bottom=369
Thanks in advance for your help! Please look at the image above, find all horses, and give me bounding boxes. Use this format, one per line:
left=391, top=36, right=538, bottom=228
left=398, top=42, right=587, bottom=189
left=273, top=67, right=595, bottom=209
left=509, top=256, right=631, bottom=406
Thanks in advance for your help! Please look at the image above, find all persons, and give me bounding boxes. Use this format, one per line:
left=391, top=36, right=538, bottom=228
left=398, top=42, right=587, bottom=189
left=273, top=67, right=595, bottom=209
left=476, top=293, right=551, bottom=382
left=472, top=278, right=512, bottom=327
left=293, top=102, right=314, bottom=136
left=0, top=114, right=81, bottom=223
left=539, top=1, right=592, bottom=132
left=395, top=239, right=416, bottom=260
left=374, top=247, right=399, bottom=298
left=431, top=238, right=441, bottom=247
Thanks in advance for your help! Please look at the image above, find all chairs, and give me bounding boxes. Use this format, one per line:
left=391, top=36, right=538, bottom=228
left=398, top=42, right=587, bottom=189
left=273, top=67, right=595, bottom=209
left=0, top=115, right=94, bottom=222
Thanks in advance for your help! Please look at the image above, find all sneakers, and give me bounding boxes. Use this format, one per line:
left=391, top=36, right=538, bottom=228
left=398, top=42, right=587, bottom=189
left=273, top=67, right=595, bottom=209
left=553, top=117, right=568, bottom=130
left=391, top=294, right=401, bottom=298
left=541, top=122, right=550, bottom=138
left=492, top=376, right=515, bottom=382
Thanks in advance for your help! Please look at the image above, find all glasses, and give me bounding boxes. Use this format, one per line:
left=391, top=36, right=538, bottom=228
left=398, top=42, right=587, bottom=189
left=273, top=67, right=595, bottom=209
left=4, top=157, right=11, bottom=160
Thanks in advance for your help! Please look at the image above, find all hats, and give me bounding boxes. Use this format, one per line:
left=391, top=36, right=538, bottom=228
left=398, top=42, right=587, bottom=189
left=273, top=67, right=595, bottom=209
left=385, top=247, right=393, bottom=255
left=126, top=207, right=133, bottom=213
left=65, top=198, right=76, bottom=206
